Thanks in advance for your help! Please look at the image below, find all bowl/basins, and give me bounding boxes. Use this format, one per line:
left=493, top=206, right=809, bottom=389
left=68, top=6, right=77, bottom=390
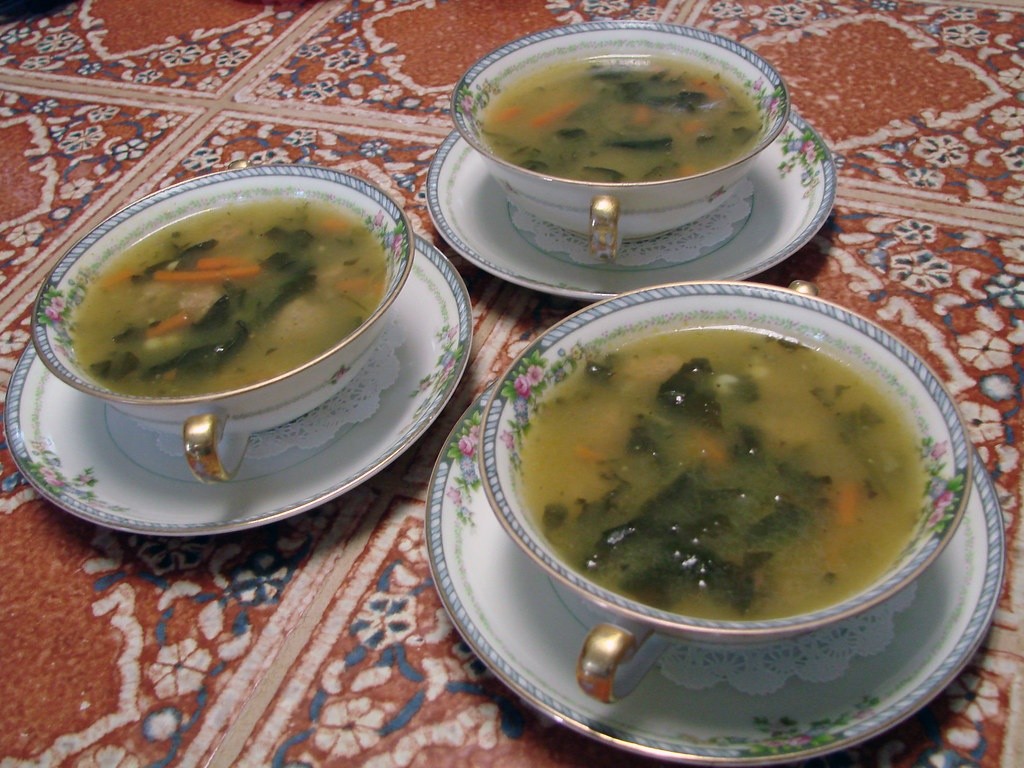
left=476, top=282, right=975, bottom=703
left=451, top=19, right=792, bottom=256
left=29, top=163, right=415, bottom=487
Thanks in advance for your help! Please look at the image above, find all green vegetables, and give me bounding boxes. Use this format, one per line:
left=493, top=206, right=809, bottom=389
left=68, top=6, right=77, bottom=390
left=537, top=352, right=897, bottom=617
left=89, top=222, right=321, bottom=397
left=479, top=61, right=758, bottom=183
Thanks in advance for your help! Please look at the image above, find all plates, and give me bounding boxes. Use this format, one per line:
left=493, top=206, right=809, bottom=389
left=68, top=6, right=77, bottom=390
left=424, top=111, right=837, bottom=299
left=4, top=234, right=476, bottom=538
left=423, top=382, right=1006, bottom=766
